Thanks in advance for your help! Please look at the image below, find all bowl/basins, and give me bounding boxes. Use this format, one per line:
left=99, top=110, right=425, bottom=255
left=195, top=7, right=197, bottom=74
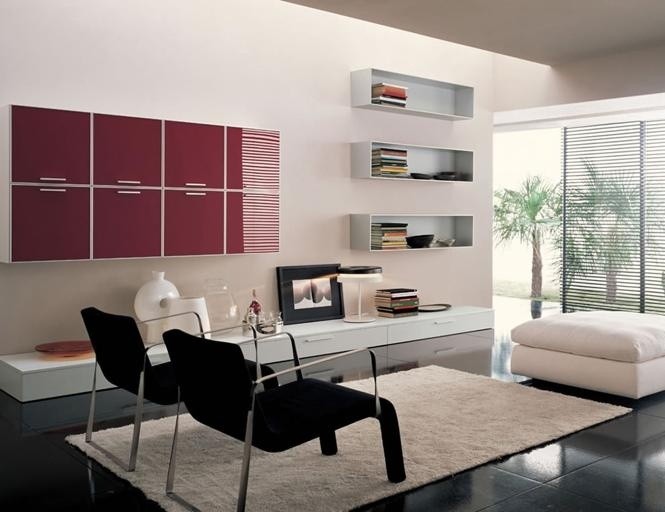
left=404, top=233, right=434, bottom=248
left=439, top=238, right=456, bottom=246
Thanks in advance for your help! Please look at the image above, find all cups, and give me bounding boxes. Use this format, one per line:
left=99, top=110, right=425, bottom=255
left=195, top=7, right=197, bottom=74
left=258, top=311, right=266, bottom=323
left=268, top=310, right=283, bottom=323
left=242, top=321, right=255, bottom=336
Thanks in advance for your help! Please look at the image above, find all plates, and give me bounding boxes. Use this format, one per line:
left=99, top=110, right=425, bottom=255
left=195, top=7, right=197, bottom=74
left=410, top=172, right=431, bottom=179
left=418, top=304, right=452, bottom=312
left=433, top=171, right=457, bottom=180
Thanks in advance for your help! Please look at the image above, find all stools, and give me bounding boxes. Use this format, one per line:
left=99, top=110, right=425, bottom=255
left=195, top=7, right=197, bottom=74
left=509, top=311, right=665, bottom=400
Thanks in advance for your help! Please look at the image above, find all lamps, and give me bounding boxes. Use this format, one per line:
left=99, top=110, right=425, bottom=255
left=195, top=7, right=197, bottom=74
left=337, top=264, right=385, bottom=323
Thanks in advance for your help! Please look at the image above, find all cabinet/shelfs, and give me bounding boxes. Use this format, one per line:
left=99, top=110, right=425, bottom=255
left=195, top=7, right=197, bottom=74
left=1, top=304, right=495, bottom=403
left=1, top=105, right=93, bottom=262
left=163, top=120, right=226, bottom=257
left=351, top=69, right=476, bottom=120
left=351, top=140, right=474, bottom=182
left=350, top=214, right=475, bottom=250
left=226, top=127, right=280, bottom=255
left=92, top=113, right=164, bottom=260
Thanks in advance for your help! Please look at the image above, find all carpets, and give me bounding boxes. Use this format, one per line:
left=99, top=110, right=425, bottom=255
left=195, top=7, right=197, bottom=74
left=63, top=365, right=631, bottom=511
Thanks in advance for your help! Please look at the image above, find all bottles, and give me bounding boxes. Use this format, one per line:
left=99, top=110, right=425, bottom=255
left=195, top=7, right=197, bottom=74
left=198, top=278, right=240, bottom=333
left=247, top=288, right=264, bottom=314
left=247, top=307, right=256, bottom=325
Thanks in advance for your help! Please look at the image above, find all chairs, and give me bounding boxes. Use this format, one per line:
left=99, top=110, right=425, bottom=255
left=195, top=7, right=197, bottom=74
left=162, top=328, right=407, bottom=512
left=80, top=306, right=280, bottom=472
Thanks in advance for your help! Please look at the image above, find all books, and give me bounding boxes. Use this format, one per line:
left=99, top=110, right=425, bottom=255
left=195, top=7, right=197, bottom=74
left=370, top=82, right=420, bottom=318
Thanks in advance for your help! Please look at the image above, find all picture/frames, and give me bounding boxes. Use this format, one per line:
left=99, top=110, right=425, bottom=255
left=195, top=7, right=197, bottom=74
left=276, top=264, right=346, bottom=325
